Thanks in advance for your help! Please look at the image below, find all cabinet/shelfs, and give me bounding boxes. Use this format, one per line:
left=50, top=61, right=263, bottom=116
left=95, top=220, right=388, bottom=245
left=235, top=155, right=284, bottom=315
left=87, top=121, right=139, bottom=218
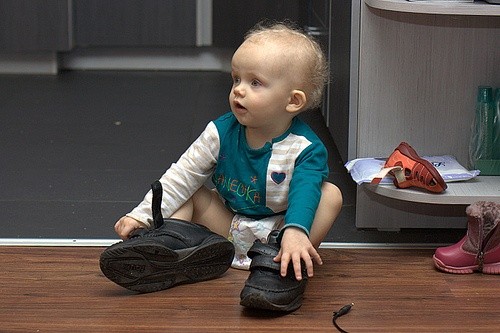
left=0, top=0, right=499, bottom=231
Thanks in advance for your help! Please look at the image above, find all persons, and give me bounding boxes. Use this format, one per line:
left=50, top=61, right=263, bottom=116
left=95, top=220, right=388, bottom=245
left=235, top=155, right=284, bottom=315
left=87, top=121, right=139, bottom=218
left=100, top=23, right=343, bottom=311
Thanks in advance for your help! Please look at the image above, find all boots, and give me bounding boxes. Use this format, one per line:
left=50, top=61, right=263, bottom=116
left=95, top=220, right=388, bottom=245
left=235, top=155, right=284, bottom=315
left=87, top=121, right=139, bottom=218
left=432, top=201, right=500, bottom=274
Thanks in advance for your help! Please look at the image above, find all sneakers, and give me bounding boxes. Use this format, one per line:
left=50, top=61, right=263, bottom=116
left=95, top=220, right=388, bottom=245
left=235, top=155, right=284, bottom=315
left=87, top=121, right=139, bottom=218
left=371, top=141, right=448, bottom=193
left=239, top=228, right=307, bottom=314
left=99, top=180, right=236, bottom=294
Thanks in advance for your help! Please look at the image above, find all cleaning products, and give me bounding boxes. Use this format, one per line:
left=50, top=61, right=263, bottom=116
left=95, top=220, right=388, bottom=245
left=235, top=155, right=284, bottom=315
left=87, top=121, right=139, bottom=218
left=468, top=86, right=500, bottom=176
